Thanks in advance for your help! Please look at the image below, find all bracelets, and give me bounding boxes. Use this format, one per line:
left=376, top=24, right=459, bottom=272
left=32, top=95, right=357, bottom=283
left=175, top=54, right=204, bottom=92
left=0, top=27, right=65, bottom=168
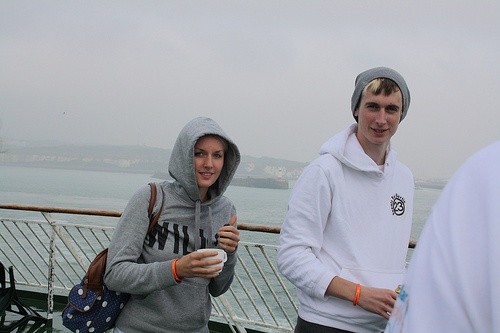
left=352, top=284, right=361, bottom=304
left=172, top=260, right=181, bottom=282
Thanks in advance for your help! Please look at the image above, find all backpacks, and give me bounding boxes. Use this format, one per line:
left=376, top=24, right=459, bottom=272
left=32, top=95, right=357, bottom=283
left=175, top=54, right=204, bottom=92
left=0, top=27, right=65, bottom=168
left=61, top=182, right=167, bottom=333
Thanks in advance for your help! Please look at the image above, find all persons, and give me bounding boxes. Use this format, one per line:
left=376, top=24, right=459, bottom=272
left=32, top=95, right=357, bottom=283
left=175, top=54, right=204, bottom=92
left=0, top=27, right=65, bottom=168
left=383, top=138, right=500, bottom=333
left=103, top=117, right=240, bottom=333
left=276, top=67, right=415, bottom=333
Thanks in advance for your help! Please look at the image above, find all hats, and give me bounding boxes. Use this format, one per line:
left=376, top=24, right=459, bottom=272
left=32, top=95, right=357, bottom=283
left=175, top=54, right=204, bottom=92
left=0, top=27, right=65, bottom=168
left=351, top=66, right=411, bottom=124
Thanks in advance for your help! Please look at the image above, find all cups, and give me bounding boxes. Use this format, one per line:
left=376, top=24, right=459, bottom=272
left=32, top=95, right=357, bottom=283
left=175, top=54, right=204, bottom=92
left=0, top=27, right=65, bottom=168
left=197, top=249, right=227, bottom=273
